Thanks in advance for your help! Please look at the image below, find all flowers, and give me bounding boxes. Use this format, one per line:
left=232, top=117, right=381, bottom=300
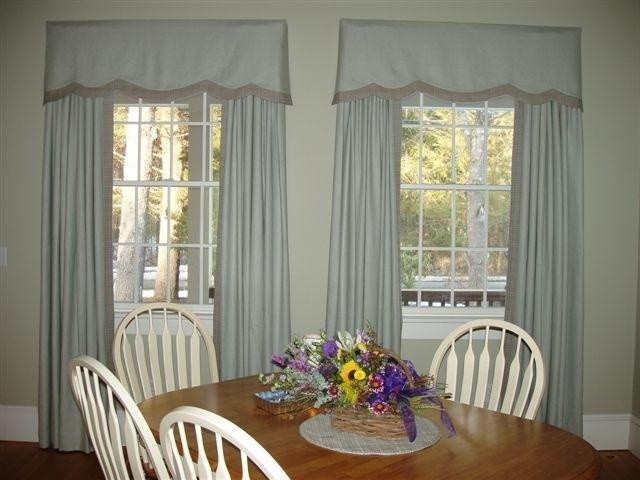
left=256, top=319, right=457, bottom=443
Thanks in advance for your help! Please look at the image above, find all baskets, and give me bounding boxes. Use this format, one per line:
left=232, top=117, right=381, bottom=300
left=331, top=349, right=415, bottom=440
left=252, top=391, right=317, bottom=416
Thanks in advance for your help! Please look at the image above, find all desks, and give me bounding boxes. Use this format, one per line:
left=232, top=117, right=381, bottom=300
left=135, top=371, right=603, bottom=480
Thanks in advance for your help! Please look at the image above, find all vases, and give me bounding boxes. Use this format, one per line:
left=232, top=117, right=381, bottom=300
left=330, top=397, right=411, bottom=439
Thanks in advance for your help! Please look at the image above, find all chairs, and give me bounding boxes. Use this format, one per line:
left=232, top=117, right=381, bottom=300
left=155, top=404, right=293, bottom=478
left=69, top=354, right=171, bottom=479
left=111, top=302, right=219, bottom=479
left=426, top=319, right=546, bottom=420
